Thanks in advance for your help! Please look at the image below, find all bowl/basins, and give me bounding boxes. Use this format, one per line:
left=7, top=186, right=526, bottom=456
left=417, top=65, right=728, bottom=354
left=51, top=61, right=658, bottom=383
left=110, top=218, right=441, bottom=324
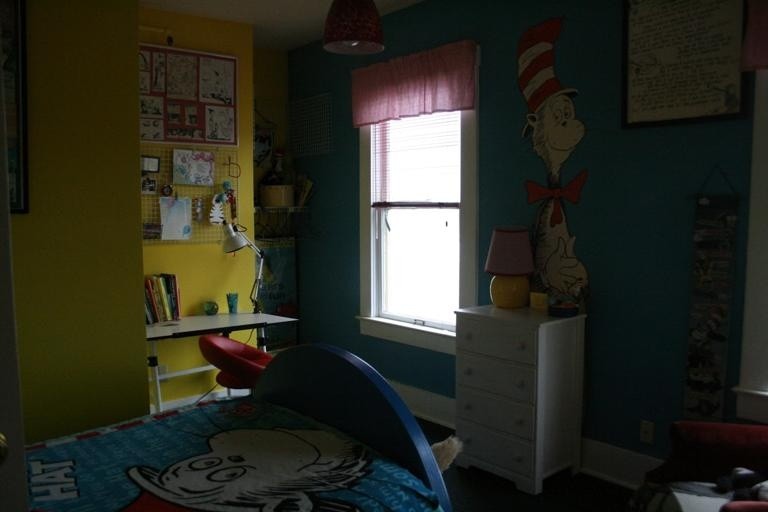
left=259, top=185, right=295, bottom=207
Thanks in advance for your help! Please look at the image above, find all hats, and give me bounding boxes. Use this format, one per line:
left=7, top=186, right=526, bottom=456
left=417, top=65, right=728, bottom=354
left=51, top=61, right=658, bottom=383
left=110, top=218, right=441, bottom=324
left=513, top=14, right=581, bottom=138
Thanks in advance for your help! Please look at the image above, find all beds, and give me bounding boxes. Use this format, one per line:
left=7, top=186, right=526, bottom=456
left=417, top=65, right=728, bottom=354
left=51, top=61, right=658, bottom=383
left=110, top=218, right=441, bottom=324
left=22, top=339, right=455, bottom=512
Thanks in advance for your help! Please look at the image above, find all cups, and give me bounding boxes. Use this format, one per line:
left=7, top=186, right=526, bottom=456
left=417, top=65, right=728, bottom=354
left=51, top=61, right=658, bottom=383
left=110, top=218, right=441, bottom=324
left=227, top=294, right=239, bottom=313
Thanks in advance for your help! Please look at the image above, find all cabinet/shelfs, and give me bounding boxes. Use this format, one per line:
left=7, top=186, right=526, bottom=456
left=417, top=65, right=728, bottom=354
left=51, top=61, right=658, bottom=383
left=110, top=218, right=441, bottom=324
left=452, top=305, right=588, bottom=498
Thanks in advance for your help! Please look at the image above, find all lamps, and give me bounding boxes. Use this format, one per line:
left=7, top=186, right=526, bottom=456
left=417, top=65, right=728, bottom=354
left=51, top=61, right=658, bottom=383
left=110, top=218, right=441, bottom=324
left=222, top=222, right=266, bottom=315
left=483, top=225, right=537, bottom=310
left=321, top=0, right=384, bottom=57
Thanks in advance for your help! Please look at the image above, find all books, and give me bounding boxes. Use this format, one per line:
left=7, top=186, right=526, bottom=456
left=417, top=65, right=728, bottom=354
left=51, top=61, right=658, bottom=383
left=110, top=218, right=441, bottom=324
left=141, top=271, right=179, bottom=325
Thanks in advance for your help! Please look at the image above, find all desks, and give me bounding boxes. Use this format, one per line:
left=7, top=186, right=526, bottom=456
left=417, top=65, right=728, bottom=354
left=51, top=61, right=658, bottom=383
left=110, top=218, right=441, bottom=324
left=145, top=311, right=300, bottom=414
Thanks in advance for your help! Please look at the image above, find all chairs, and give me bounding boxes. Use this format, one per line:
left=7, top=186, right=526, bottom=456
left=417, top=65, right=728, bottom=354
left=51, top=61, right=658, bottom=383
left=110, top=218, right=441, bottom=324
left=195, top=336, right=272, bottom=400
left=628, top=418, right=767, bottom=511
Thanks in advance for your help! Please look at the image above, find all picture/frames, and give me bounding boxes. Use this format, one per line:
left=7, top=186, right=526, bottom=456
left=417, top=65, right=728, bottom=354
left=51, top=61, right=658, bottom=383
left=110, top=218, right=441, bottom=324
left=0, top=1, right=31, bottom=215
left=617, top=0, right=750, bottom=131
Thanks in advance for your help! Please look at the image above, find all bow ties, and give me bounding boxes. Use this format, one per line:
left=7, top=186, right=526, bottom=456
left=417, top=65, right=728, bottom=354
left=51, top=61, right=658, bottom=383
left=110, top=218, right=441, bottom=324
left=526, top=168, right=588, bottom=227
